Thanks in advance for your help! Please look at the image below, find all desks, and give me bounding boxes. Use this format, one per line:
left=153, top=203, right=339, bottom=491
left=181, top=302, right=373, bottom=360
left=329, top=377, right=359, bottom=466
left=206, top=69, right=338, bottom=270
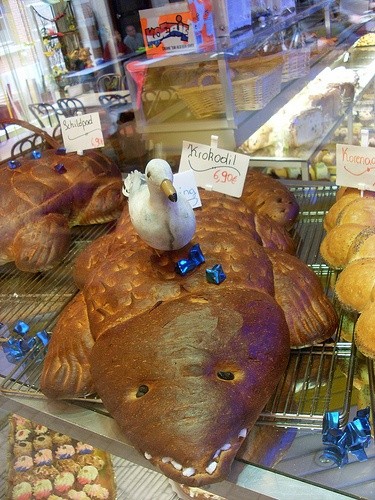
left=63, top=49, right=146, bottom=92
left=48, top=84, right=180, bottom=109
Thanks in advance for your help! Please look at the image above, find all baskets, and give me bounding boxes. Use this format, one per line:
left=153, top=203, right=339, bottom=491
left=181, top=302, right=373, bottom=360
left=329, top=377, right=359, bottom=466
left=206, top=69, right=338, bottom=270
left=176, top=60, right=286, bottom=118
left=205, top=48, right=311, bottom=80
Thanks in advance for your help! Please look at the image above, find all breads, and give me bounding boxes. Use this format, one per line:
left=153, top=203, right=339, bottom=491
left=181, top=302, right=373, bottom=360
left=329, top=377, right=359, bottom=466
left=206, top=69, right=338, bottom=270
left=241, top=73, right=375, bottom=182
left=0, top=146, right=129, bottom=272
left=38, top=172, right=340, bottom=485
left=356, top=32, right=375, bottom=46
left=320, top=183, right=375, bottom=361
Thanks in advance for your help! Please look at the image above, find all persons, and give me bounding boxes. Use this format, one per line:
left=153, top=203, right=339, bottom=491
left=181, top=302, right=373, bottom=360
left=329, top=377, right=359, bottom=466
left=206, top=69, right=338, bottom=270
left=124, top=26, right=144, bottom=53
left=104, top=30, right=126, bottom=62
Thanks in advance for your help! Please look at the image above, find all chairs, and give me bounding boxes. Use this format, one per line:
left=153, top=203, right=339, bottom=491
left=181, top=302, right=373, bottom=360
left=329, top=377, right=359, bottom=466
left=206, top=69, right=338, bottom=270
left=10, top=68, right=202, bottom=158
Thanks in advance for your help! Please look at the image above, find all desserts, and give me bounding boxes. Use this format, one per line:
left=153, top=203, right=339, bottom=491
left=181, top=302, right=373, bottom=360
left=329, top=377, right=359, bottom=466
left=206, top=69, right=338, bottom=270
left=11, top=415, right=109, bottom=500
left=180, top=485, right=230, bottom=500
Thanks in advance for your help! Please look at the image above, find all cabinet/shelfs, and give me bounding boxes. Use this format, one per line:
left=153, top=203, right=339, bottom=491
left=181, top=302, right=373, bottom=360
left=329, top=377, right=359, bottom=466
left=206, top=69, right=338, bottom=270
left=124, top=0, right=375, bottom=179
